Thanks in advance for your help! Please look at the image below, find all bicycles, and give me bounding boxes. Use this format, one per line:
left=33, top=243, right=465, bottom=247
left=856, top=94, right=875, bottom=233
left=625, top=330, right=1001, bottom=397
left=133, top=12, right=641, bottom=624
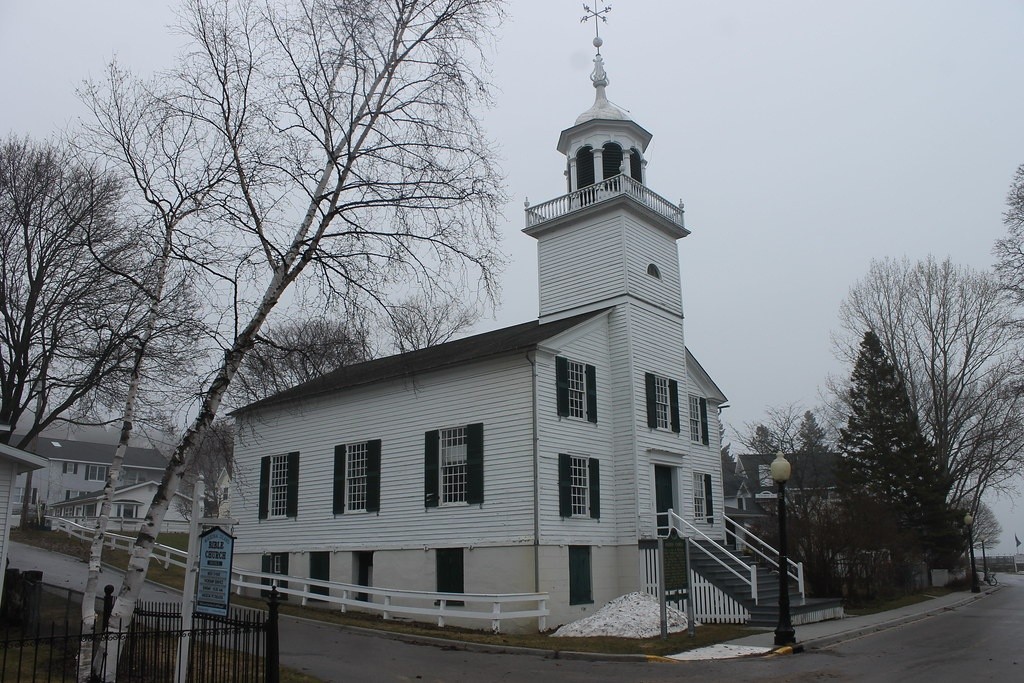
left=988, top=573, right=998, bottom=586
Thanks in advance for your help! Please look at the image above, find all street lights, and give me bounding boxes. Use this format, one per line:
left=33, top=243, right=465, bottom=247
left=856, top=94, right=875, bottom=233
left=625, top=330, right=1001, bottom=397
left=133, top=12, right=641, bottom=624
left=979, top=534, right=992, bottom=585
left=770, top=451, right=797, bottom=647
left=963, top=512, right=981, bottom=593
